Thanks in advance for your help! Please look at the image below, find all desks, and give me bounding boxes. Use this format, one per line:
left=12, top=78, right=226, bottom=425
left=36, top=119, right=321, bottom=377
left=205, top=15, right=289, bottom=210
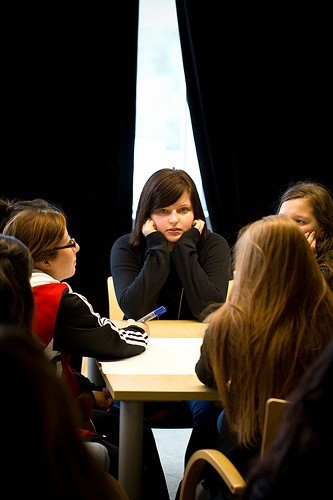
left=96, top=317, right=228, bottom=500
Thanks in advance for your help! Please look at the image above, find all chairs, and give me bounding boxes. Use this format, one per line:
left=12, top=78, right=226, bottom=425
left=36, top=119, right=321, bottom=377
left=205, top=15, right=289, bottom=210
left=179, top=398, right=297, bottom=500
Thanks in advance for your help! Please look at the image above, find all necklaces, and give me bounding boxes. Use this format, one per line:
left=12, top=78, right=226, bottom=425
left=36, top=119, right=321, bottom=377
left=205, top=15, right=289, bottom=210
left=154, top=288, right=184, bottom=319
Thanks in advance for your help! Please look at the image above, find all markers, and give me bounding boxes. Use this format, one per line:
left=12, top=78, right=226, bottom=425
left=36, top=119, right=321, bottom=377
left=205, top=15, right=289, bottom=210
left=135, top=306, right=167, bottom=325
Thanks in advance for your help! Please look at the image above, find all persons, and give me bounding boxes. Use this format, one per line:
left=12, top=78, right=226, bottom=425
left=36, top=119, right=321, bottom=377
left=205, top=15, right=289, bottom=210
left=195, top=182, right=333, bottom=489
left=108, top=168, right=231, bottom=500
left=0, top=198, right=149, bottom=500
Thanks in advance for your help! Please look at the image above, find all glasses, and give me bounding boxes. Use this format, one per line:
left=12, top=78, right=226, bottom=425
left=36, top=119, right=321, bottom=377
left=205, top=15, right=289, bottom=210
left=48, top=237, right=77, bottom=253
